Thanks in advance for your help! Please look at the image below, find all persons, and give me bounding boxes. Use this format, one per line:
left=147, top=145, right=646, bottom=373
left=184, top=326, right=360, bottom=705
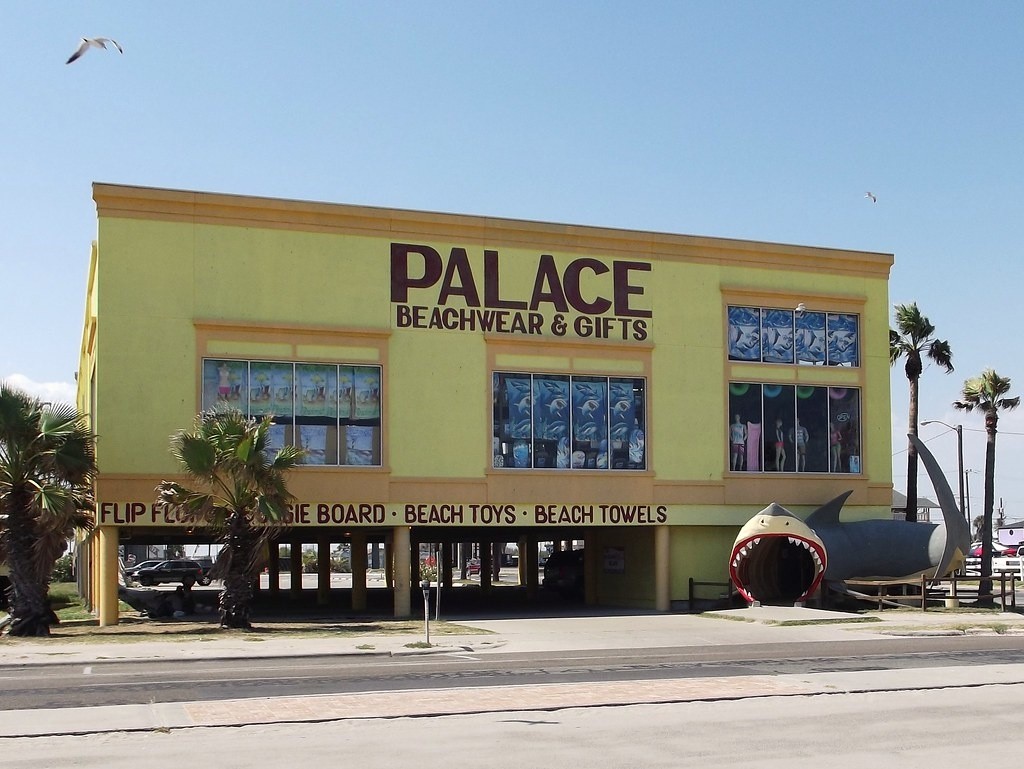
left=217, top=362, right=232, bottom=401
left=774, top=418, right=786, bottom=472
left=789, top=417, right=809, bottom=472
left=729, top=414, right=748, bottom=471
left=829, top=422, right=843, bottom=473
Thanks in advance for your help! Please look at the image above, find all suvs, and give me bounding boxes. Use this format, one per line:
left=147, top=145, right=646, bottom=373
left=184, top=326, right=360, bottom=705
left=125, top=555, right=218, bottom=586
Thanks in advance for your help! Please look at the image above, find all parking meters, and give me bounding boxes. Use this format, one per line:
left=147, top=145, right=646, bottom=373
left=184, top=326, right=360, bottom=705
left=421, top=580, right=431, bottom=643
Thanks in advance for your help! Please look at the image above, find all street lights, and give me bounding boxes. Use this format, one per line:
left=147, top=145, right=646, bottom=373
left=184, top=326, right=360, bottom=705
left=920, top=418, right=965, bottom=517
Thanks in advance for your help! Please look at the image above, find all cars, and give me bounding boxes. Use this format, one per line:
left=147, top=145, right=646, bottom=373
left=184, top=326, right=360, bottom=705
left=466, top=558, right=494, bottom=577
